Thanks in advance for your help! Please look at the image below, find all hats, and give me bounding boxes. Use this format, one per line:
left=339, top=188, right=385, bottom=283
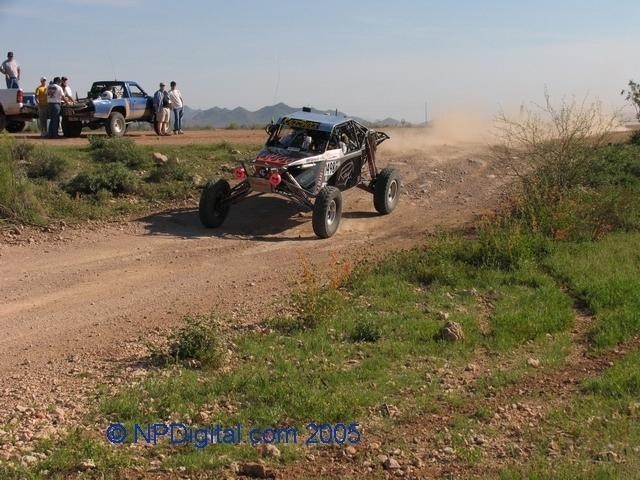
left=160, top=83, right=165, bottom=86
left=40, top=77, right=47, bottom=81
left=62, top=77, right=68, bottom=81
left=7, top=52, right=13, bottom=57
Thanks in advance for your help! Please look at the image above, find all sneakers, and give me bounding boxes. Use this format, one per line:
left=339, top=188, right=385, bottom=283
left=159, top=130, right=183, bottom=136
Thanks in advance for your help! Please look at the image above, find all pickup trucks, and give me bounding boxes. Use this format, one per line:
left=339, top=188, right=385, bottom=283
left=0, top=87, right=39, bottom=133
left=62, top=79, right=156, bottom=139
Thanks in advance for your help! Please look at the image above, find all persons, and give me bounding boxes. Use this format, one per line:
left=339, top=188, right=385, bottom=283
left=46, top=77, right=69, bottom=137
left=97, top=86, right=113, bottom=100
left=301, top=131, right=311, bottom=151
left=35, top=77, right=49, bottom=137
left=153, top=82, right=171, bottom=135
left=168, top=81, right=184, bottom=134
left=0, top=51, right=20, bottom=89
left=329, top=135, right=347, bottom=154
left=59, top=77, right=72, bottom=136
